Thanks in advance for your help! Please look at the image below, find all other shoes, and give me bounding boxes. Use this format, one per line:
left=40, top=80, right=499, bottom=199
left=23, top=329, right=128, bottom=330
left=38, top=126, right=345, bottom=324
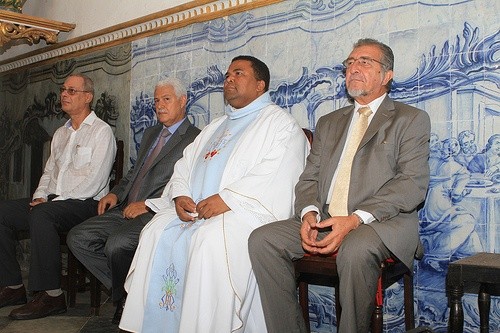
left=112, top=297, right=126, bottom=324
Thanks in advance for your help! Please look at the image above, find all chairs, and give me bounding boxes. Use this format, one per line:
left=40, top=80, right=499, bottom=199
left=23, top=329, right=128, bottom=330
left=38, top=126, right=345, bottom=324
left=59, top=129, right=414, bottom=333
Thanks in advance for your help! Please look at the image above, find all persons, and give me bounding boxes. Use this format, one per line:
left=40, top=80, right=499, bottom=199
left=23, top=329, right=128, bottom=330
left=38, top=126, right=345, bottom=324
left=0, top=72, right=118, bottom=320
left=65, top=79, right=203, bottom=323
left=139, top=55, right=311, bottom=333
left=247, top=38, right=431, bottom=333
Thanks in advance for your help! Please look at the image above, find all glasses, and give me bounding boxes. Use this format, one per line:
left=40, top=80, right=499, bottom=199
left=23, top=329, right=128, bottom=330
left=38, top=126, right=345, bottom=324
left=342, top=56, right=387, bottom=67
left=60, top=87, right=91, bottom=95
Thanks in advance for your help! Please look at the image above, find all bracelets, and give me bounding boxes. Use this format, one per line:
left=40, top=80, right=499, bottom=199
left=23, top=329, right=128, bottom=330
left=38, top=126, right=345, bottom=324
left=353, top=213, right=364, bottom=228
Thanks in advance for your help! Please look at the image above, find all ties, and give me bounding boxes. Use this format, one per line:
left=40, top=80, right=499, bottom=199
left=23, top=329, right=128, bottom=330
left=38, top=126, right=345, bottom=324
left=129, top=129, right=171, bottom=203
left=328, top=108, right=373, bottom=217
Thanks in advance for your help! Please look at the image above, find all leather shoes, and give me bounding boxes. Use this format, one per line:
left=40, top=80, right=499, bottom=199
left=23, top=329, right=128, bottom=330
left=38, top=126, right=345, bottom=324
left=8, top=291, right=67, bottom=319
left=0, top=284, right=27, bottom=307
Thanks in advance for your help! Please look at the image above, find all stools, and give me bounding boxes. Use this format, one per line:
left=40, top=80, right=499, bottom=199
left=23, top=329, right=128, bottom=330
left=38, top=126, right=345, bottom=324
left=446, top=251, right=500, bottom=333
